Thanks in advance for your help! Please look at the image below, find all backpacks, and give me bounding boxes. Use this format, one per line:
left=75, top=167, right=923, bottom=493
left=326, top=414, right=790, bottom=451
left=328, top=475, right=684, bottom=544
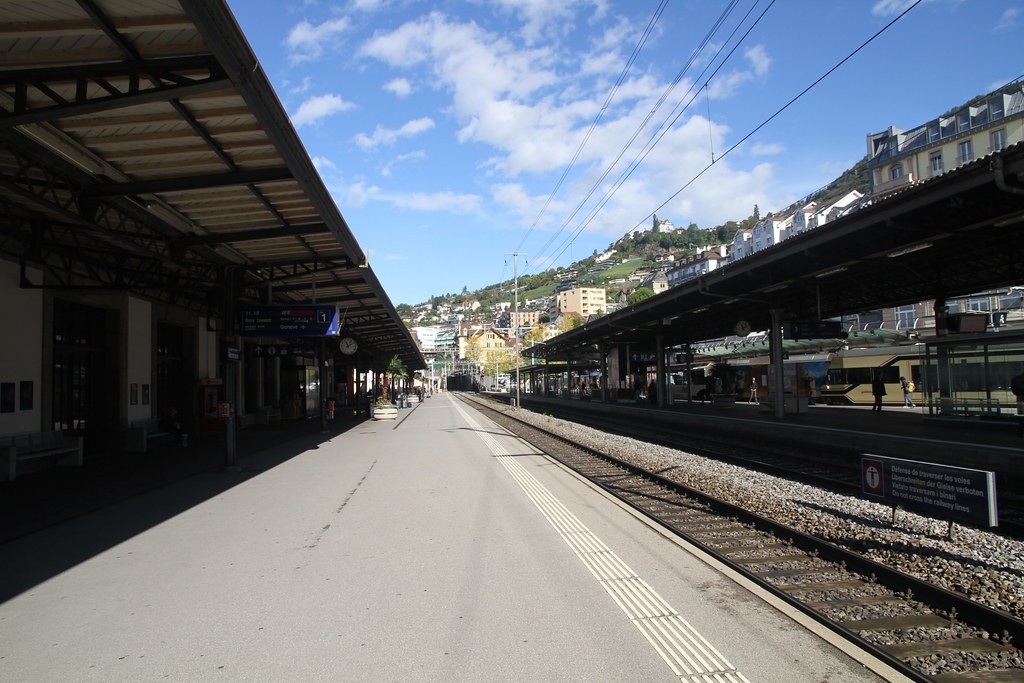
left=906, top=381, right=916, bottom=393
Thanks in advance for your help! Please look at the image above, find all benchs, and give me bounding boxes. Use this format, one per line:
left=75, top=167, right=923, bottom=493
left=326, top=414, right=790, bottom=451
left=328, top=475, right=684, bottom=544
left=258, top=409, right=281, bottom=424
left=125, top=418, right=172, bottom=453
left=0, top=429, right=83, bottom=482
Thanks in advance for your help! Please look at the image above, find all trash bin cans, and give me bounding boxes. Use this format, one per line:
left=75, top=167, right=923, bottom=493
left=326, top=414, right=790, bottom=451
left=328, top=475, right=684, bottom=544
left=326, top=398, right=336, bottom=419
left=600, top=388, right=617, bottom=402
left=370, top=399, right=375, bottom=418
left=562, top=388, right=570, bottom=399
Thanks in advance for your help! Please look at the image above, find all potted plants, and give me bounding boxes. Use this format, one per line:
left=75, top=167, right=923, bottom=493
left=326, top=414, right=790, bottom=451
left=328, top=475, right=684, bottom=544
left=373, top=354, right=409, bottom=420
left=708, top=363, right=739, bottom=409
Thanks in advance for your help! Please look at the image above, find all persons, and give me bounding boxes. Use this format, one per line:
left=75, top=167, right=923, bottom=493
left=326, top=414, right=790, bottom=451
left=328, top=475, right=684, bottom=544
left=872, top=373, right=887, bottom=410
left=571, top=380, right=598, bottom=394
left=650, top=379, right=656, bottom=403
left=900, top=377, right=916, bottom=408
left=749, top=377, right=759, bottom=404
left=697, top=389, right=714, bottom=403
left=1011, top=373, right=1024, bottom=414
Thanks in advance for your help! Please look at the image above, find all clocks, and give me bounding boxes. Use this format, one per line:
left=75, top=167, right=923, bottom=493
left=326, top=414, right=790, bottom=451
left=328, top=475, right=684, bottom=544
left=339, top=337, right=357, bottom=356
left=735, top=320, right=751, bottom=337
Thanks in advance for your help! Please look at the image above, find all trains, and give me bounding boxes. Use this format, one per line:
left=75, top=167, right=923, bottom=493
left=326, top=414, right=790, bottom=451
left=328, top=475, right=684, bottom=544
left=649, top=337, right=1024, bottom=408
